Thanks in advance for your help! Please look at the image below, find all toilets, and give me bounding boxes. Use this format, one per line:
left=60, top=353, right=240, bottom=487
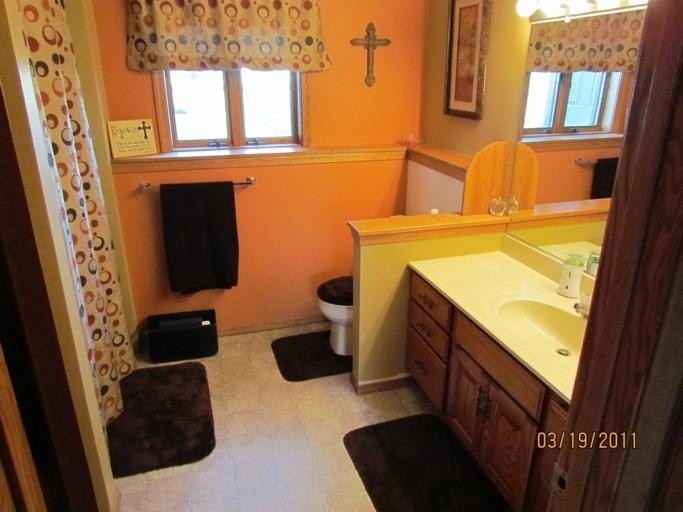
left=316, top=276, right=353, bottom=356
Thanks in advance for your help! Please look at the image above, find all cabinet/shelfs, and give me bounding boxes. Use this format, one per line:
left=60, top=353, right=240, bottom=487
left=405, top=270, right=570, bottom=510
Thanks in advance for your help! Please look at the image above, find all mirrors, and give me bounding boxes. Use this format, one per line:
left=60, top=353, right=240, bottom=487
left=501, top=9, right=647, bottom=299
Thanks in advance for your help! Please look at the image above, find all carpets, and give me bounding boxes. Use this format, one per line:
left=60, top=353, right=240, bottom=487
left=343, top=413, right=508, bottom=511
left=270, top=330, right=353, bottom=383
left=105, top=362, right=216, bottom=479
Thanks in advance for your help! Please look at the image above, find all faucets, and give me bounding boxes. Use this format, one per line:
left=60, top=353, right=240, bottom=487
left=574, top=303, right=589, bottom=318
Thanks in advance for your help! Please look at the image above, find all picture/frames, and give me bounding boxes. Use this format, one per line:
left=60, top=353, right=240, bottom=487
left=443, top=0, right=490, bottom=118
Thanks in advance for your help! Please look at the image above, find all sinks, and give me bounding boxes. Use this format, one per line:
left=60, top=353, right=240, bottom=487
left=498, top=299, right=588, bottom=366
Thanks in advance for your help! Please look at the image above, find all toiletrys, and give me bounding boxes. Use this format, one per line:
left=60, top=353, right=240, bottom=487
left=556, top=257, right=585, bottom=298
left=586, top=250, right=601, bottom=275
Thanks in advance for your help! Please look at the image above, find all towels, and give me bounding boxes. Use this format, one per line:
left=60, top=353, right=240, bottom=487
left=160, top=181, right=238, bottom=293
